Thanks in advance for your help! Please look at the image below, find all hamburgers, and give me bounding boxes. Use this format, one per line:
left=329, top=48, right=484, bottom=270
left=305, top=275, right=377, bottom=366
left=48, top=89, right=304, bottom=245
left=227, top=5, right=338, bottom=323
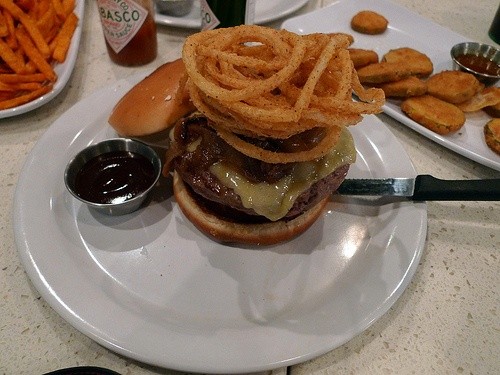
left=108, top=25, right=384, bottom=242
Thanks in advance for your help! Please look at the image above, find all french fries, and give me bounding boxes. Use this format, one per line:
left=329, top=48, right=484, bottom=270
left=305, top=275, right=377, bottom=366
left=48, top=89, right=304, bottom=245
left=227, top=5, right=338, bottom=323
left=0, top=0, right=80, bottom=110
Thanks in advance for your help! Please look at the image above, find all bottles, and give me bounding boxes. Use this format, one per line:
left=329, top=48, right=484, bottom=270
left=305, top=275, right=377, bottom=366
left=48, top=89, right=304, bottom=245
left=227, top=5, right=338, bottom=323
left=200, top=0, right=247, bottom=28
left=95, top=0, right=157, bottom=66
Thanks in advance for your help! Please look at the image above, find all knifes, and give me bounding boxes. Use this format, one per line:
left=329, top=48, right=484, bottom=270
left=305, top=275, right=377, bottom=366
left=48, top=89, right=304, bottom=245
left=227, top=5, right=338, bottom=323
left=336, top=173, right=500, bottom=201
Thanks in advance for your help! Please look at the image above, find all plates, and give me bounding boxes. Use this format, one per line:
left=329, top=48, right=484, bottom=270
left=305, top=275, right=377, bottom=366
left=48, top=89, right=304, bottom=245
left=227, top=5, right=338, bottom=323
left=279, top=1, right=499, bottom=173
left=450, top=38, right=500, bottom=86
left=15, top=53, right=429, bottom=374
left=156, top=0, right=310, bottom=27
left=0, top=0, right=88, bottom=121
left=63, top=138, right=164, bottom=218
